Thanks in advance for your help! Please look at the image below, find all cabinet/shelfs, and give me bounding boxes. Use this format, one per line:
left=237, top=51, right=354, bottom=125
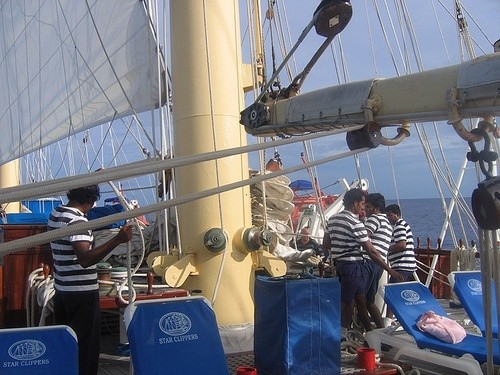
left=0, top=225, right=53, bottom=312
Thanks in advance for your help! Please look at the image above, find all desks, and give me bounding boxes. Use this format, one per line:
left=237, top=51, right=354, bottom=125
left=254, top=274, right=342, bottom=375
left=100, top=277, right=187, bottom=344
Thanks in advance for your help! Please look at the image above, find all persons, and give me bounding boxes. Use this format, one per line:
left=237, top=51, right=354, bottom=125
left=291, top=228, right=321, bottom=256
left=323, top=188, right=417, bottom=350
left=48, top=184, right=134, bottom=375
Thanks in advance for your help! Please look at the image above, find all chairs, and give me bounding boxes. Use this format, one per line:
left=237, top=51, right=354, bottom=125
left=364, top=281, right=500, bottom=375
left=447, top=270, right=498, bottom=339
left=0, top=325, right=78, bottom=375
left=124, top=296, right=229, bottom=375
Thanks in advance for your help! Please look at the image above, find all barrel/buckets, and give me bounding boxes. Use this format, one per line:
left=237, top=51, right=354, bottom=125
left=237, top=366, right=256, bottom=374
left=358, top=349, right=375, bottom=370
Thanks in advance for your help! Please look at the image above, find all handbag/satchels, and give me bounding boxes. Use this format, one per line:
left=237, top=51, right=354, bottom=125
left=416, top=310, right=466, bottom=345
